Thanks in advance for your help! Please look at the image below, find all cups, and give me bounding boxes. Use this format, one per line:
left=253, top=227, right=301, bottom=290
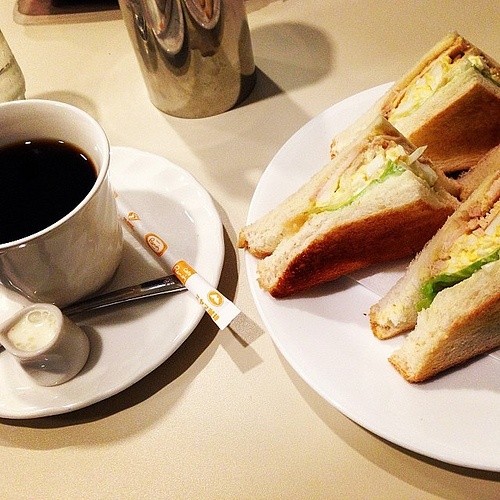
left=118, top=0, right=257, bottom=119
left=0, top=29, right=25, bottom=100
left=0, top=303, right=90, bottom=387
left=0, top=98, right=122, bottom=308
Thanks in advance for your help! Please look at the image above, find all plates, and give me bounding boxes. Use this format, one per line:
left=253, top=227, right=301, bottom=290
left=0, top=145, right=225, bottom=419
left=245, top=81, right=500, bottom=471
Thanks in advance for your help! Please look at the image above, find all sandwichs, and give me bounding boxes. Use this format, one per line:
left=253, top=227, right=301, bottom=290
left=369, top=166, right=500, bottom=384
left=329, top=30, right=500, bottom=172
left=237, top=114, right=469, bottom=297
